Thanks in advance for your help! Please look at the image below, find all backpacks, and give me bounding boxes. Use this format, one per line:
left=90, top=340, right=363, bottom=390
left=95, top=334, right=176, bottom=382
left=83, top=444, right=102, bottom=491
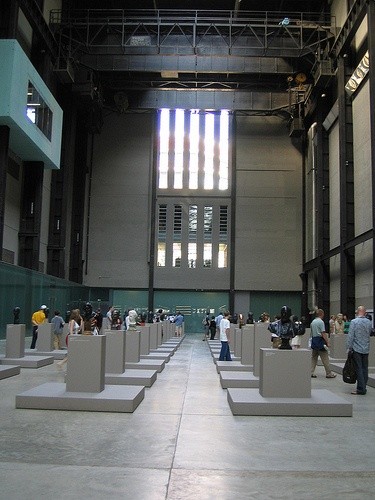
left=202, top=318, right=207, bottom=326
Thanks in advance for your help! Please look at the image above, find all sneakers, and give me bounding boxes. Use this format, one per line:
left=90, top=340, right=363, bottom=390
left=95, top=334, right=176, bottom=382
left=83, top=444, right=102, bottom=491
left=311, top=373, right=317, bottom=378
left=351, top=388, right=367, bottom=395
left=326, top=373, right=335, bottom=378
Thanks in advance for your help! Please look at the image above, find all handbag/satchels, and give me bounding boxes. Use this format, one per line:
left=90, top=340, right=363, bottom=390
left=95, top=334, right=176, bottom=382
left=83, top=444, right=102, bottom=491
left=342, top=351, right=356, bottom=384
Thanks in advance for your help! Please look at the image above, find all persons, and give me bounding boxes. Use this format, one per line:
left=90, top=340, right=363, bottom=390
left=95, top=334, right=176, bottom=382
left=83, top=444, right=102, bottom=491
left=309, top=309, right=336, bottom=378
left=31, top=305, right=349, bottom=361
left=346, top=307, right=372, bottom=396
